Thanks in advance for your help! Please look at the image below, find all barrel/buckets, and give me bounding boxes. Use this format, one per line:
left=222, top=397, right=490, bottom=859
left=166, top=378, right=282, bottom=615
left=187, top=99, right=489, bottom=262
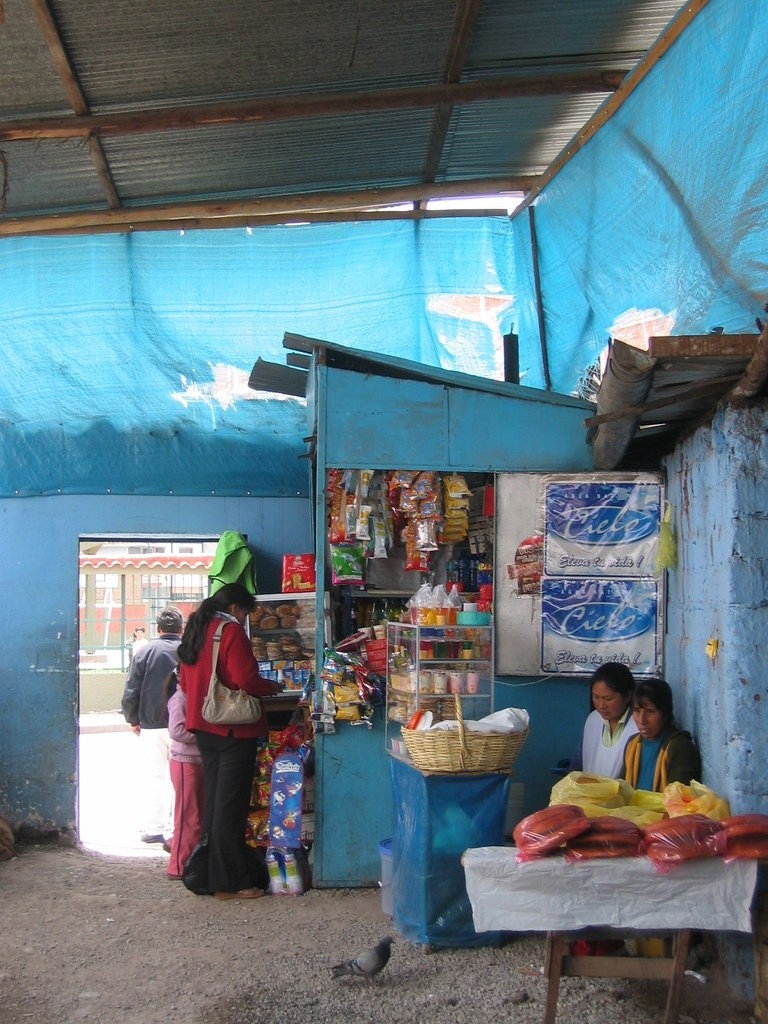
left=377, top=839, right=396, bottom=915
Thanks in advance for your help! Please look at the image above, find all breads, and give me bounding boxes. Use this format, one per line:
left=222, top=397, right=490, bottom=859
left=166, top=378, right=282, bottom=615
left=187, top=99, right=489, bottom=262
left=251, top=636, right=313, bottom=662
left=250, top=604, right=301, bottom=629
left=513, top=806, right=768, bottom=861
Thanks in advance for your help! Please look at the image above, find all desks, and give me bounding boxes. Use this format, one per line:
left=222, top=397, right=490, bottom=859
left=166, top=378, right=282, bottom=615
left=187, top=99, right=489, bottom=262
left=461, top=847, right=762, bottom=1024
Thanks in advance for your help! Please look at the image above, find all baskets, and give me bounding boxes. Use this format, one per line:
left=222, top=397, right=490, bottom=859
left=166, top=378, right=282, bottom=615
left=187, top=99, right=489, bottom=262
left=401, top=694, right=528, bottom=773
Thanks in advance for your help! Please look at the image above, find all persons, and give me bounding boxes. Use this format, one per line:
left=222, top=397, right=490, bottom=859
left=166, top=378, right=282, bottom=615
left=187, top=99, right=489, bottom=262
left=542, top=661, right=702, bottom=958
left=116, top=582, right=285, bottom=901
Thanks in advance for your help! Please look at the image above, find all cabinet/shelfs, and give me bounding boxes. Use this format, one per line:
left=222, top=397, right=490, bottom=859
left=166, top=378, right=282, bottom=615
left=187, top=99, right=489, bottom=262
left=247, top=589, right=516, bottom=953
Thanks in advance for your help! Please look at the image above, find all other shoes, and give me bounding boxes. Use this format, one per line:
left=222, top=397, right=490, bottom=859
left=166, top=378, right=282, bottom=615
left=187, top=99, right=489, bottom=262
left=163, top=845, right=170, bottom=852
left=141, top=835, right=163, bottom=843
left=168, top=874, right=182, bottom=880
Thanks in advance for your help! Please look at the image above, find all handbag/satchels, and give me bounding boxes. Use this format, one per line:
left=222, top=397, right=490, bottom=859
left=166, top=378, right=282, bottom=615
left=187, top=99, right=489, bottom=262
left=202, top=620, right=261, bottom=724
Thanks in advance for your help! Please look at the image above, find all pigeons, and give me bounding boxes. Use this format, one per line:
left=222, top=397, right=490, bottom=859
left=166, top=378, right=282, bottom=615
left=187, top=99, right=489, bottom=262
left=329, top=936, right=397, bottom=990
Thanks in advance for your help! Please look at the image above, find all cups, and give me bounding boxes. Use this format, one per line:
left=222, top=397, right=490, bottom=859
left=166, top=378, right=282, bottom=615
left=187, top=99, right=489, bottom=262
left=420, top=671, right=431, bottom=694
left=420, top=650, right=428, bottom=658
left=462, top=649, right=474, bottom=658
left=467, top=673, right=479, bottom=693
left=450, top=673, right=463, bottom=693
left=434, top=673, right=447, bottom=694
left=411, top=671, right=416, bottom=693
left=464, top=603, right=477, bottom=611
left=390, top=674, right=400, bottom=688
left=400, top=677, right=407, bottom=691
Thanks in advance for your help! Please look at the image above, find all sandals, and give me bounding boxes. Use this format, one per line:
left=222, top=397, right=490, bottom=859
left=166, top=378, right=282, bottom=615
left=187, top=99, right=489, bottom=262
left=216, top=887, right=266, bottom=900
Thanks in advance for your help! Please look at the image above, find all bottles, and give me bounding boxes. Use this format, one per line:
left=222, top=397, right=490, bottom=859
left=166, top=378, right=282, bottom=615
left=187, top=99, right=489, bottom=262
left=447, top=561, right=453, bottom=583
left=350, top=601, right=358, bottom=636
left=468, top=560, right=476, bottom=592
left=432, top=585, right=450, bottom=627
left=266, top=845, right=287, bottom=896
left=400, top=600, right=408, bottom=615
left=447, top=585, right=461, bottom=625
left=416, top=584, right=432, bottom=626
left=368, top=601, right=379, bottom=626
left=457, top=560, right=465, bottom=582
left=391, top=600, right=400, bottom=622
left=380, top=599, right=391, bottom=626
left=282, top=846, right=304, bottom=894
